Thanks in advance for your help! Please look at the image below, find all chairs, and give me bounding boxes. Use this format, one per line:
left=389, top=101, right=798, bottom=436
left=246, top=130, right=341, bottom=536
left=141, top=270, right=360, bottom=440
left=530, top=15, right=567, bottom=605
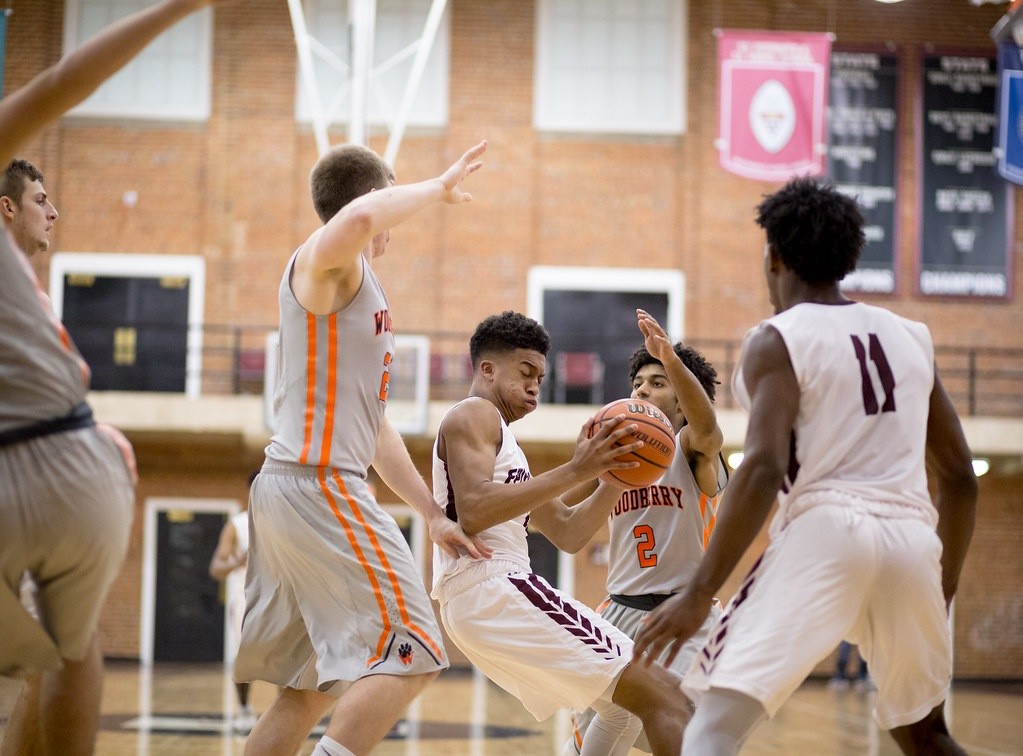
left=212, top=345, right=610, bottom=403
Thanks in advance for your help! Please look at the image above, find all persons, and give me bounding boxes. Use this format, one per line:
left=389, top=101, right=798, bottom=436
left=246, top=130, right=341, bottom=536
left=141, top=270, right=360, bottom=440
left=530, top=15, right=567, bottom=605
left=432, top=311, right=695, bottom=756
left=633, top=178, right=980, bottom=756
left=0, top=0, right=242, bottom=756
left=233, top=140, right=494, bottom=756
left=561, top=309, right=729, bottom=756
left=0, top=159, right=58, bottom=259
left=211, top=472, right=257, bottom=733
left=831, top=641, right=868, bottom=689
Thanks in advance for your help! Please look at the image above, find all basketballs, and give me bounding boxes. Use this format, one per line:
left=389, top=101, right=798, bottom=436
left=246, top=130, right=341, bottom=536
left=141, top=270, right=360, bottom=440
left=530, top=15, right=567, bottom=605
left=587, top=398, right=676, bottom=491
